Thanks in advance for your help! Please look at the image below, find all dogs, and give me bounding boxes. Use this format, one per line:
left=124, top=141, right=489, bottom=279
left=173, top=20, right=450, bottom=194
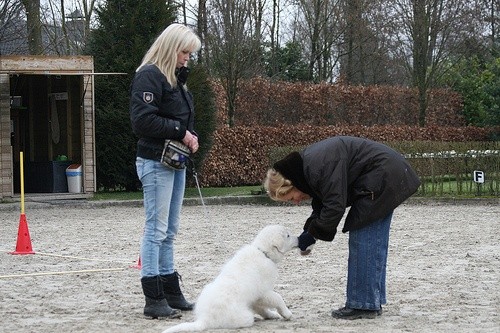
left=161, top=224, right=299, bottom=333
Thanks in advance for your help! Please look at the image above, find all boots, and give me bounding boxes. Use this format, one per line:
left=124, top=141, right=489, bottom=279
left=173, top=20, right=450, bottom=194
left=141, top=275, right=183, bottom=319
left=160, top=269, right=195, bottom=311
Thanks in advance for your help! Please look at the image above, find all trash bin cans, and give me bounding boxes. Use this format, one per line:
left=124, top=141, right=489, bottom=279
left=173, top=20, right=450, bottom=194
left=65, top=165, right=82, bottom=193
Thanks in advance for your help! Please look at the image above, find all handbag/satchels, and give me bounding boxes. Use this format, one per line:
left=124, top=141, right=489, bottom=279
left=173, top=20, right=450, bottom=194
left=161, top=139, right=191, bottom=171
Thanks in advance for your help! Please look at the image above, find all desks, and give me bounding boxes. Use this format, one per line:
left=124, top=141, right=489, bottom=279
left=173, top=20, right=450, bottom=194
left=24, top=160, right=71, bottom=192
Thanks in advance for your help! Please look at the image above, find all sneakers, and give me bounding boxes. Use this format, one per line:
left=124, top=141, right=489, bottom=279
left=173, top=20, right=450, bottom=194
left=332, top=306, right=382, bottom=320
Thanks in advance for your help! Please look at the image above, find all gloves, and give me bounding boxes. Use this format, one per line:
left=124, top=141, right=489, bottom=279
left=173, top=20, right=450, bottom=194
left=298, top=231, right=316, bottom=251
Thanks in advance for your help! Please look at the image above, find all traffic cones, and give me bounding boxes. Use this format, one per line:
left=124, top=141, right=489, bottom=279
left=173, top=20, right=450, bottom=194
left=7, top=213, right=36, bottom=256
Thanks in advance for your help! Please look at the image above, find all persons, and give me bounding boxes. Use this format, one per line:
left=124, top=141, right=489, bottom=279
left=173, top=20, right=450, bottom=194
left=265, top=136, right=421, bottom=319
left=128, top=22, right=202, bottom=322
